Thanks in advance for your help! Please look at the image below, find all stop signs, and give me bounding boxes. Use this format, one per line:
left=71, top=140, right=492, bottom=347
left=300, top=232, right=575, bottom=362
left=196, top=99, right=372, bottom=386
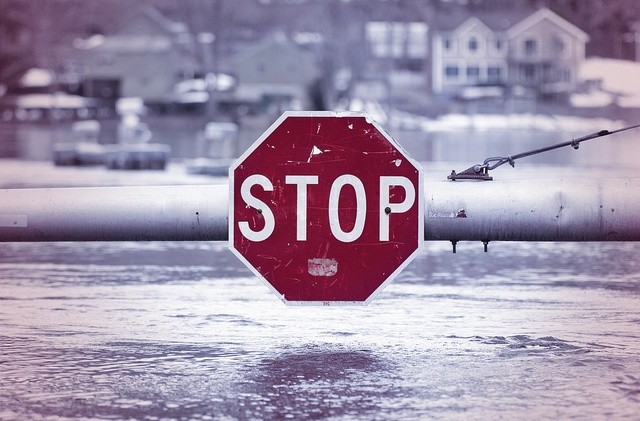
left=228, top=111, right=425, bottom=306
